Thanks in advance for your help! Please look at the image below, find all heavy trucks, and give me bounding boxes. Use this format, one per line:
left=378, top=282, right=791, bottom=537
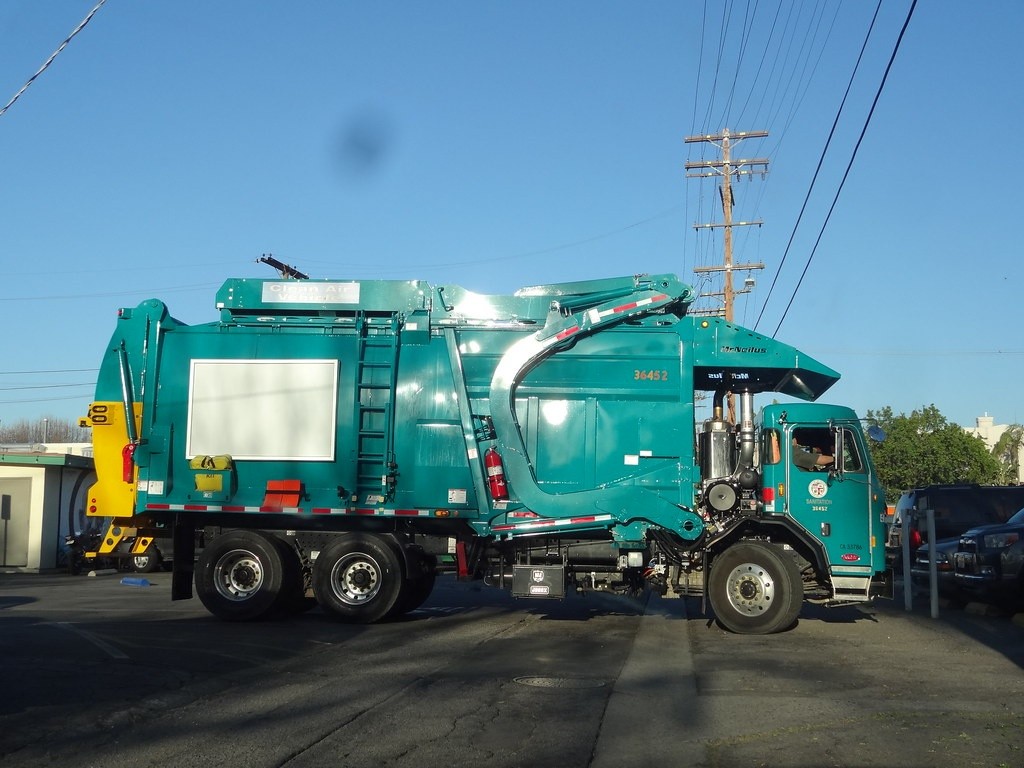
left=74, top=257, right=896, bottom=631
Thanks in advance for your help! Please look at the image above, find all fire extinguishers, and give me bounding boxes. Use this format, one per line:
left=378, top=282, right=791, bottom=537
left=484, top=445, right=508, bottom=500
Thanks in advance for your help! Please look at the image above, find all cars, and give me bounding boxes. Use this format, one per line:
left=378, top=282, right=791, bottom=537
left=909, top=536, right=961, bottom=604
left=884, top=482, right=1024, bottom=573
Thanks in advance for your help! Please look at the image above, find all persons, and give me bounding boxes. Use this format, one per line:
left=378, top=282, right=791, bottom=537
left=792, top=432, right=835, bottom=473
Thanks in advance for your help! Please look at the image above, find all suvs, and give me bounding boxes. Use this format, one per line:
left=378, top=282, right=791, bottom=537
left=949, top=506, right=1024, bottom=605
